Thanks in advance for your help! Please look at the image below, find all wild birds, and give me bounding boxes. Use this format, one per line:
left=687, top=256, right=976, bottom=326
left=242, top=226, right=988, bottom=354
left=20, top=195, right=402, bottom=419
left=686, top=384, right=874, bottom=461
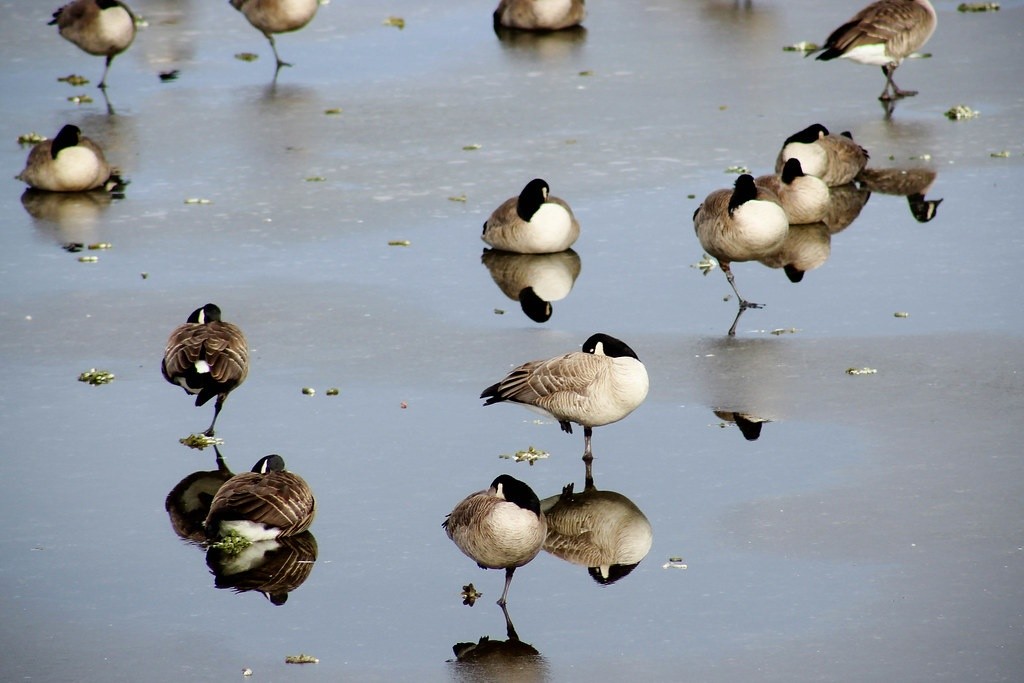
left=441, top=473, right=548, bottom=601
left=774, top=119, right=870, bottom=187
left=162, top=303, right=249, bottom=439
left=165, top=439, right=236, bottom=548
left=851, top=166, right=944, bottom=223
left=480, top=333, right=649, bottom=458
left=227, top=0, right=322, bottom=68
left=21, top=189, right=124, bottom=253
left=492, top=0, right=589, bottom=31
left=756, top=223, right=831, bottom=284
left=44, top=1, right=136, bottom=85
left=536, top=458, right=653, bottom=585
left=779, top=1, right=938, bottom=100
left=755, top=157, right=832, bottom=221
left=203, top=454, right=316, bottom=542
left=694, top=174, right=788, bottom=307
left=15, top=125, right=125, bottom=192
left=480, top=178, right=580, bottom=254
left=206, top=530, right=318, bottom=606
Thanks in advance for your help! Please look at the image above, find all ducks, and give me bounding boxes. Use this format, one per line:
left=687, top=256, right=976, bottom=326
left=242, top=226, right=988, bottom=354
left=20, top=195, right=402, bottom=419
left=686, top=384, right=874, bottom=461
left=480, top=249, right=582, bottom=323
